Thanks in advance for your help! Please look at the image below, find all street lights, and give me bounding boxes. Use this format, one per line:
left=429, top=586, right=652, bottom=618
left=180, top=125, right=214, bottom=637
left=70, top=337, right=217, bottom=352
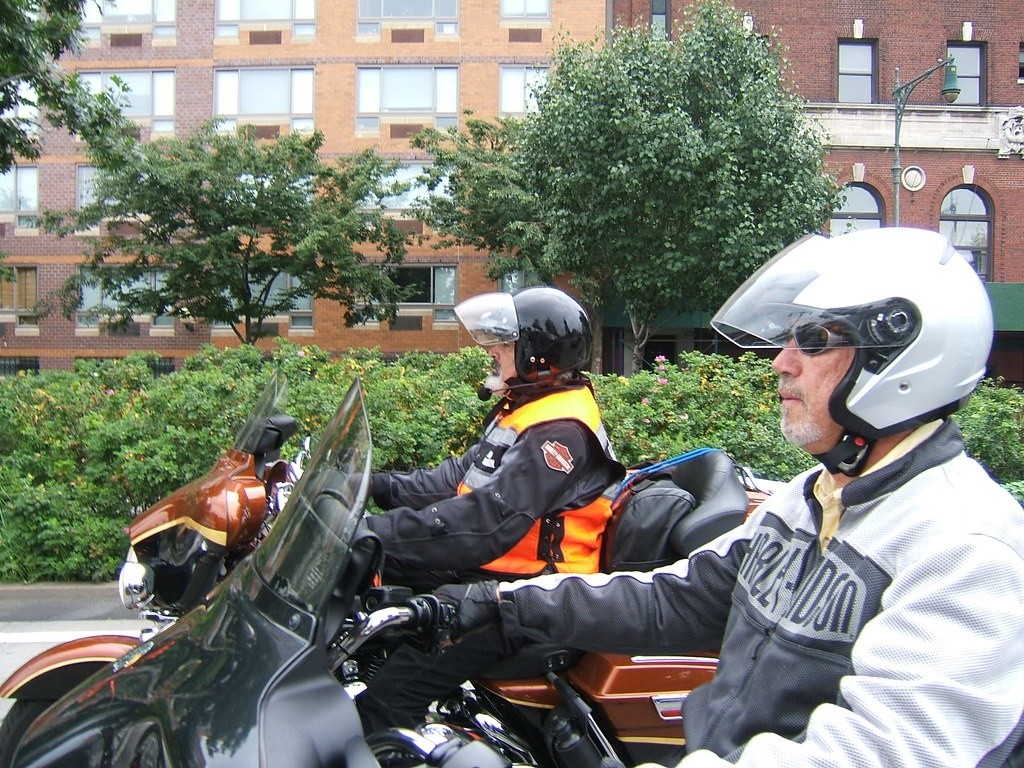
left=892, top=52, right=962, bottom=227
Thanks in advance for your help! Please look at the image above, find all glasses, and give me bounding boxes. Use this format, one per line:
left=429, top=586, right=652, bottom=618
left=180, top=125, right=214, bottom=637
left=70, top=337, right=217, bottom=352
left=782, top=325, right=856, bottom=357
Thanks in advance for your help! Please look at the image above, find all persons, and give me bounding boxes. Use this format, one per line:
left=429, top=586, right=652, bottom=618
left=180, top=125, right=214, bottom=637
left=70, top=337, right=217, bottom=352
left=282, top=286, right=628, bottom=744
left=409, top=226, right=1024, bottom=768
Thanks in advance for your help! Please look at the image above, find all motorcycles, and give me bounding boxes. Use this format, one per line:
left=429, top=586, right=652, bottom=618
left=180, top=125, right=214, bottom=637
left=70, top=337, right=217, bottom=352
left=1, top=370, right=631, bottom=768
left=118, top=368, right=787, bottom=736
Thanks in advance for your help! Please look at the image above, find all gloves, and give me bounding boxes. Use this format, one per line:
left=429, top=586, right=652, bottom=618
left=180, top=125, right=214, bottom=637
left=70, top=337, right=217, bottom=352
left=432, top=578, right=499, bottom=642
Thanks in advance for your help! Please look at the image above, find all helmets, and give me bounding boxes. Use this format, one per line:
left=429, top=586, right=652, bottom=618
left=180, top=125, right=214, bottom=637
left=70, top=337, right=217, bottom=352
left=754, top=226, right=995, bottom=438
left=473, top=286, right=592, bottom=382
